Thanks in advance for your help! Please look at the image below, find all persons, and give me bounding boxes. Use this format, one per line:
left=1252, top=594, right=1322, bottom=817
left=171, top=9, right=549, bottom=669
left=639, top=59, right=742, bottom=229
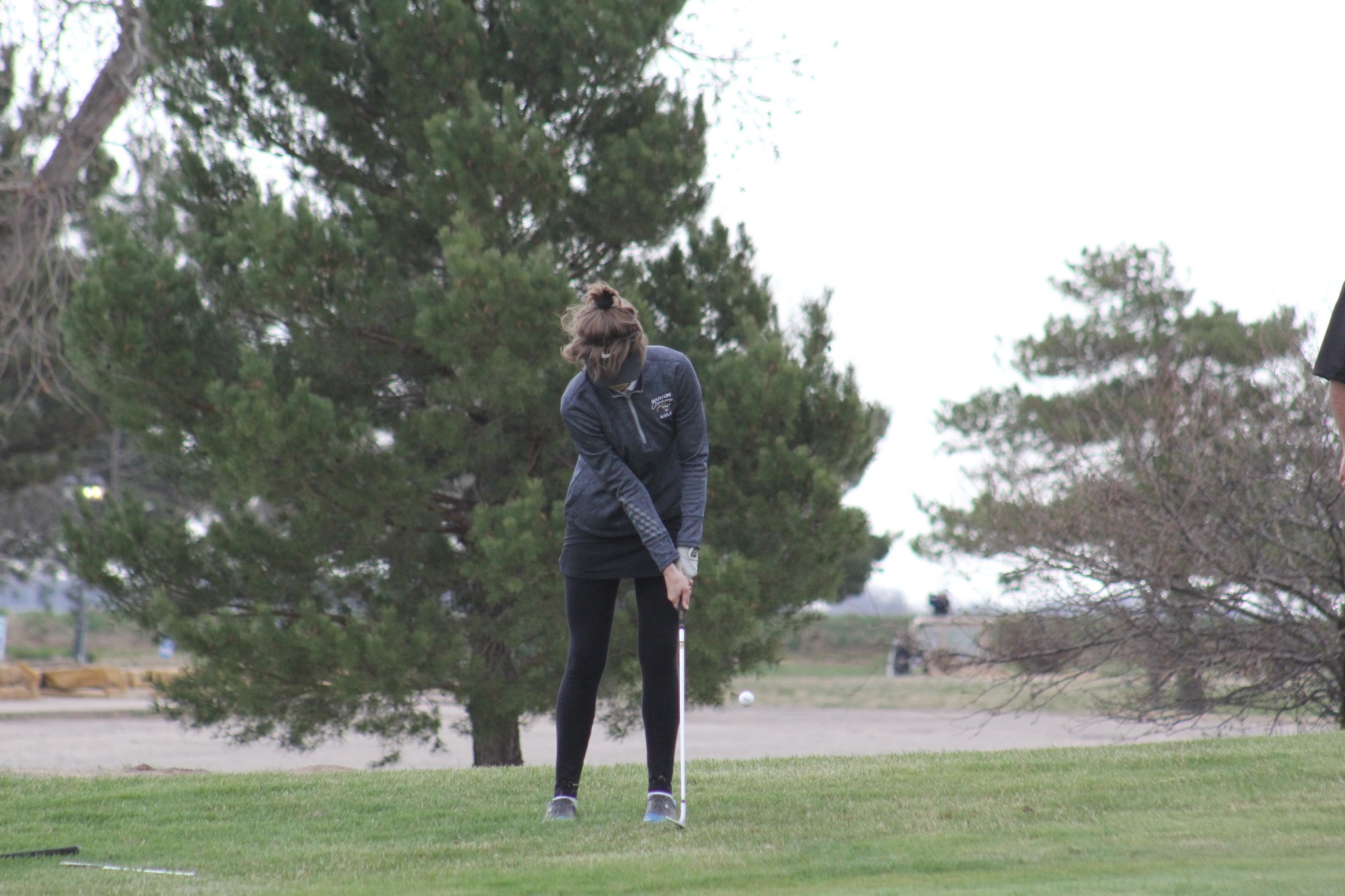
left=545, top=283, right=710, bottom=828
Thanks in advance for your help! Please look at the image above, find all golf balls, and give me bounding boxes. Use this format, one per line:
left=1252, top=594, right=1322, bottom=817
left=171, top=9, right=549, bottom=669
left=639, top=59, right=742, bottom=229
left=738, top=691, right=754, bottom=707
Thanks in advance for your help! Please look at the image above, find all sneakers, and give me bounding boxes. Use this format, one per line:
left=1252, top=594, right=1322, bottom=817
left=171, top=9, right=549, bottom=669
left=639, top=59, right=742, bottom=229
left=542, top=796, right=577, bottom=820
left=643, top=791, right=677, bottom=822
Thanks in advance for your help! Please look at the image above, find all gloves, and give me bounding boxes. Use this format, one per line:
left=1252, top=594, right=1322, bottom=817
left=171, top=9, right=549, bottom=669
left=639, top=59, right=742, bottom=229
left=675, top=546, right=698, bottom=578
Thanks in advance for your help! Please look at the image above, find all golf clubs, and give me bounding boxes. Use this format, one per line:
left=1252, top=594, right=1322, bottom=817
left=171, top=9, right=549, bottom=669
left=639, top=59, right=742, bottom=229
left=664, top=591, right=688, bottom=831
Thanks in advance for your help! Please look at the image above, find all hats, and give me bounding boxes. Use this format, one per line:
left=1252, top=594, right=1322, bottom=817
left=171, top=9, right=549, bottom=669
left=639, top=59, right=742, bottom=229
left=584, top=340, right=642, bottom=387
